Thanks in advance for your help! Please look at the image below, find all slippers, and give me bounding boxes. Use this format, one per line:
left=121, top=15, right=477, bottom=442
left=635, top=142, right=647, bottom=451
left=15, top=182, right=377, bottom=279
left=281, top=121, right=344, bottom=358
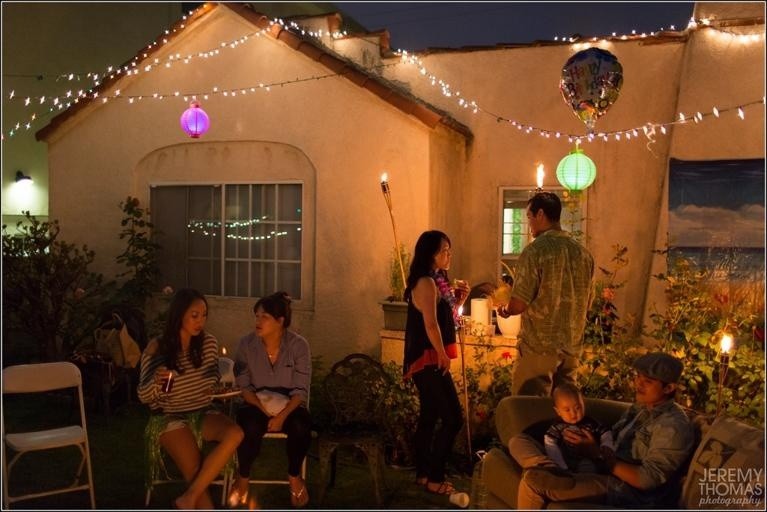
left=425, top=481, right=456, bottom=495
left=228, top=481, right=247, bottom=508
left=289, top=480, right=309, bottom=507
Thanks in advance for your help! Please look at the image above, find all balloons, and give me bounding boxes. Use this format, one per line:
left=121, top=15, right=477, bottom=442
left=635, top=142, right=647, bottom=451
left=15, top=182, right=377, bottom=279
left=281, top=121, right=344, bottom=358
left=558, top=46, right=624, bottom=134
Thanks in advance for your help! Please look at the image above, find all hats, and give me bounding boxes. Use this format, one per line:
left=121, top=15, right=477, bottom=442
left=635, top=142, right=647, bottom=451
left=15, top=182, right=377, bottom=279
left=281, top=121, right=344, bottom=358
left=633, top=353, right=684, bottom=383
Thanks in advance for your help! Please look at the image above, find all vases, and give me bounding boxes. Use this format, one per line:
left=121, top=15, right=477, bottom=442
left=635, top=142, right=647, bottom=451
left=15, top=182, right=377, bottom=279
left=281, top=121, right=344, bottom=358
left=470, top=297, right=522, bottom=340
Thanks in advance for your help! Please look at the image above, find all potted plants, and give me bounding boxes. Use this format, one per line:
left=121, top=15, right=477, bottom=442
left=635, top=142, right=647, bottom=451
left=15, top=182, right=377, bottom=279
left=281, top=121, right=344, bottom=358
left=376, top=246, right=411, bottom=333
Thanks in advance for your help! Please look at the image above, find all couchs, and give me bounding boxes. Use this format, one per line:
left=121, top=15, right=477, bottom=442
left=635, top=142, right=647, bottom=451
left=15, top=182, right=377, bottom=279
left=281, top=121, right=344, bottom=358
left=469, top=395, right=766, bottom=509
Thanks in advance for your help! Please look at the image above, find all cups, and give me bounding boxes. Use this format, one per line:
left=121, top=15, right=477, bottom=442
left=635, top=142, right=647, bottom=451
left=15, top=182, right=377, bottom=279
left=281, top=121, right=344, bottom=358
left=454, top=279, right=465, bottom=301
left=161, top=371, right=175, bottom=394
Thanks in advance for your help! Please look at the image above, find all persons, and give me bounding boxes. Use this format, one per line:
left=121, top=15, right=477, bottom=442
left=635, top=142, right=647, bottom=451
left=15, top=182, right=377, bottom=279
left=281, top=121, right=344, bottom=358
left=227, top=290, right=311, bottom=511
left=497, top=191, right=595, bottom=396
left=400, top=227, right=463, bottom=497
left=545, top=382, right=612, bottom=477
left=135, top=287, right=246, bottom=510
left=515, top=353, right=702, bottom=510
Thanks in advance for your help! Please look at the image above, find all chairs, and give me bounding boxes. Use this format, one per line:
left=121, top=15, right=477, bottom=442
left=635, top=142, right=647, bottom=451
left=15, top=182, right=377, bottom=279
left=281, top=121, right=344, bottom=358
left=321, top=354, right=423, bottom=504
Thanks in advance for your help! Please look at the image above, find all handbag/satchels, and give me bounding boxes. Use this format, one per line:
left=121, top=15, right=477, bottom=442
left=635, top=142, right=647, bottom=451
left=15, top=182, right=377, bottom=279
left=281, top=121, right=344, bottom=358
left=95, top=312, right=140, bottom=368
